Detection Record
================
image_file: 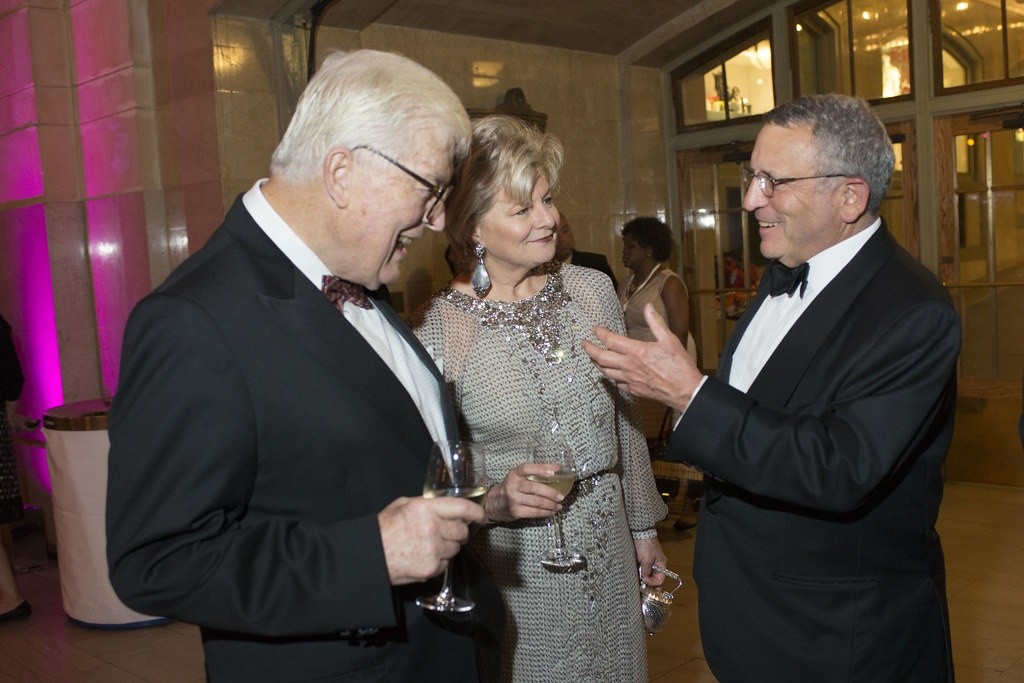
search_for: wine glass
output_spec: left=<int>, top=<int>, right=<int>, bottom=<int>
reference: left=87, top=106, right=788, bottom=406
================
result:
left=525, top=436, right=583, bottom=566
left=415, top=439, right=487, bottom=612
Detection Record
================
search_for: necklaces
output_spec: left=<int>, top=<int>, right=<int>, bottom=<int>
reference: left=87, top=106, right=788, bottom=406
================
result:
left=630, top=283, right=636, bottom=292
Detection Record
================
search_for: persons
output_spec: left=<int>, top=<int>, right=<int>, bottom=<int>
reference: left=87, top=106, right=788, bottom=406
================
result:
left=106, top=49, right=505, bottom=683
left=556, top=211, right=618, bottom=291
left=582, top=94, right=961, bottom=683
left=0, top=312, right=40, bottom=623
left=620, top=217, right=697, bottom=440
left=408, top=114, right=660, bottom=683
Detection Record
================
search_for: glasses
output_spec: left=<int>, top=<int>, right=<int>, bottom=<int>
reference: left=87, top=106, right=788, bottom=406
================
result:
left=349, top=145, right=454, bottom=223
left=740, top=165, right=851, bottom=198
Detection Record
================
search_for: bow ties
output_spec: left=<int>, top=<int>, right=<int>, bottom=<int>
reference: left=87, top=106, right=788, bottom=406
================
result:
left=322, top=275, right=374, bottom=313
left=769, top=262, right=808, bottom=299
